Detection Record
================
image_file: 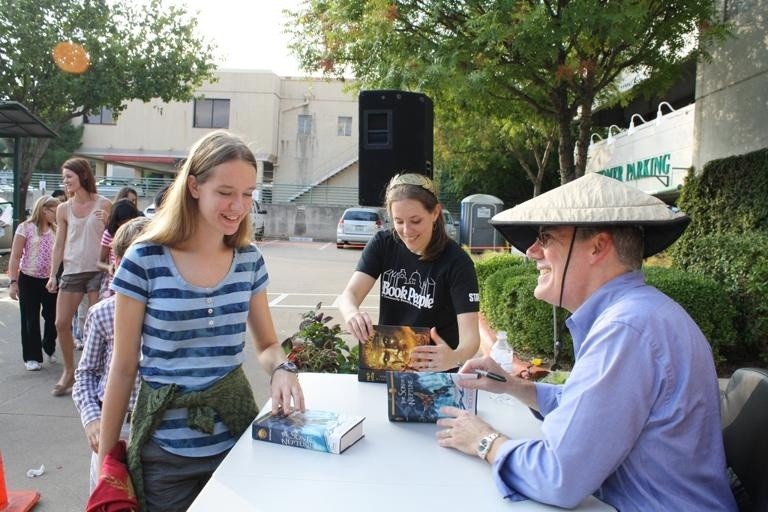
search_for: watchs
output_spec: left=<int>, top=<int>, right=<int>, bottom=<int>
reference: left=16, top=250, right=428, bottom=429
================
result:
left=476, top=433, right=499, bottom=462
left=269, top=362, right=297, bottom=383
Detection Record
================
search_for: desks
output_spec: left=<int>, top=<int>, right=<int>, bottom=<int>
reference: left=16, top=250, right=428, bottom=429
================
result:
left=182, top=370, right=621, bottom=511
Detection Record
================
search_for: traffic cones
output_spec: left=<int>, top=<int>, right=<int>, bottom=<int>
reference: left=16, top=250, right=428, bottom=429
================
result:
left=0, top=447, right=44, bottom=512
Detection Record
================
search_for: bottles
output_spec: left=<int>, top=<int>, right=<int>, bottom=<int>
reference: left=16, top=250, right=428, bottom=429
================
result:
left=489, top=331, right=515, bottom=404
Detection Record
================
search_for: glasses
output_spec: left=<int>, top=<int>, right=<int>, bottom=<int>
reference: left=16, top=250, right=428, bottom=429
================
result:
left=536, top=227, right=600, bottom=248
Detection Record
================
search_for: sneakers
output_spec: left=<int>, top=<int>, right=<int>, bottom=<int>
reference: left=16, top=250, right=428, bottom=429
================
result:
left=23, top=338, right=85, bottom=371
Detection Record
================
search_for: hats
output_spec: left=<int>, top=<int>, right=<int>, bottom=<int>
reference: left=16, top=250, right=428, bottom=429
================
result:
left=488, top=170, right=691, bottom=258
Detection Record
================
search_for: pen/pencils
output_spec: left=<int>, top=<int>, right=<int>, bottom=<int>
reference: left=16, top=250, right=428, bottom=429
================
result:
left=456, top=362, right=507, bottom=382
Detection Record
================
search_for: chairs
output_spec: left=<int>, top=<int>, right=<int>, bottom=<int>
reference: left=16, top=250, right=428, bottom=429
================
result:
left=715, top=366, right=766, bottom=511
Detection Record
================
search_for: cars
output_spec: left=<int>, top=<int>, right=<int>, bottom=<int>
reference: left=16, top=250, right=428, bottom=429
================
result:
left=0, top=197, right=33, bottom=255
left=335, top=206, right=388, bottom=250
left=0, top=183, right=38, bottom=192
left=143, top=200, right=157, bottom=219
left=440, top=208, right=459, bottom=243
left=95, top=178, right=143, bottom=195
left=249, top=200, right=269, bottom=240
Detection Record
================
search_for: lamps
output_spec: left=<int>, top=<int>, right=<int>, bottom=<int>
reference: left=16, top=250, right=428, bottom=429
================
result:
left=586, top=100, right=692, bottom=150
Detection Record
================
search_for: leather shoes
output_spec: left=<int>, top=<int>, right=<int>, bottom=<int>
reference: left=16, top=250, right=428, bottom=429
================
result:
left=51, top=377, right=76, bottom=395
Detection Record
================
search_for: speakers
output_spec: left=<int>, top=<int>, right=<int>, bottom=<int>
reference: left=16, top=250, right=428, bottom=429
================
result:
left=359, top=90, right=434, bottom=207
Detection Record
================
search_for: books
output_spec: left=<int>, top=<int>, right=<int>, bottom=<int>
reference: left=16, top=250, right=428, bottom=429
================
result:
left=252, top=406, right=366, bottom=455
left=357, top=324, right=432, bottom=384
left=385, top=372, right=477, bottom=422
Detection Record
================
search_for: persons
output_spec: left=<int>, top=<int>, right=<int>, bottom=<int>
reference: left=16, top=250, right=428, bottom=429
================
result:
left=70, top=217, right=151, bottom=493
left=8, top=156, right=168, bottom=396
left=435, top=171, right=738, bottom=512
left=98, top=130, right=306, bottom=511
left=334, top=173, right=481, bottom=374
left=362, top=326, right=430, bottom=373
